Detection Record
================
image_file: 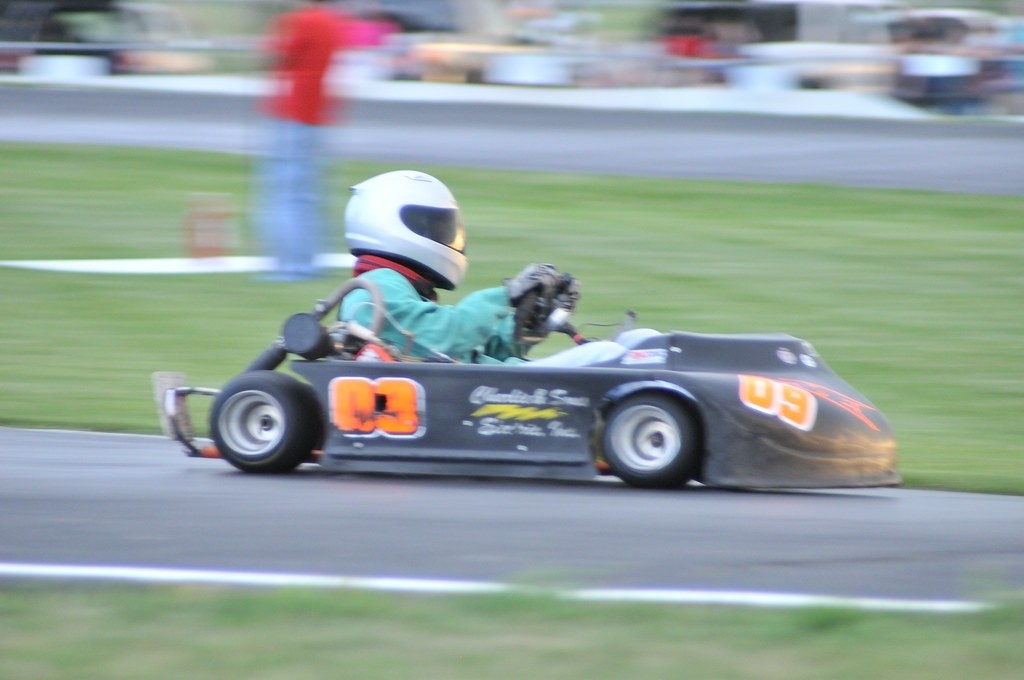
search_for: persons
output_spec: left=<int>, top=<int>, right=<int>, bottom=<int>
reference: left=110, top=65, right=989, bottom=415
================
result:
left=252, top=0, right=397, bottom=281
left=335, top=169, right=581, bottom=365
left=874, top=16, right=1024, bottom=115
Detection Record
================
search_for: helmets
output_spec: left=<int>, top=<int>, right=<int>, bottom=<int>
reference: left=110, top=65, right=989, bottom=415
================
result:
left=342, top=167, right=472, bottom=292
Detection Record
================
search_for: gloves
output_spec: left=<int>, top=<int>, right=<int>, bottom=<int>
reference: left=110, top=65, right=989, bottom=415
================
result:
left=505, top=261, right=581, bottom=309
